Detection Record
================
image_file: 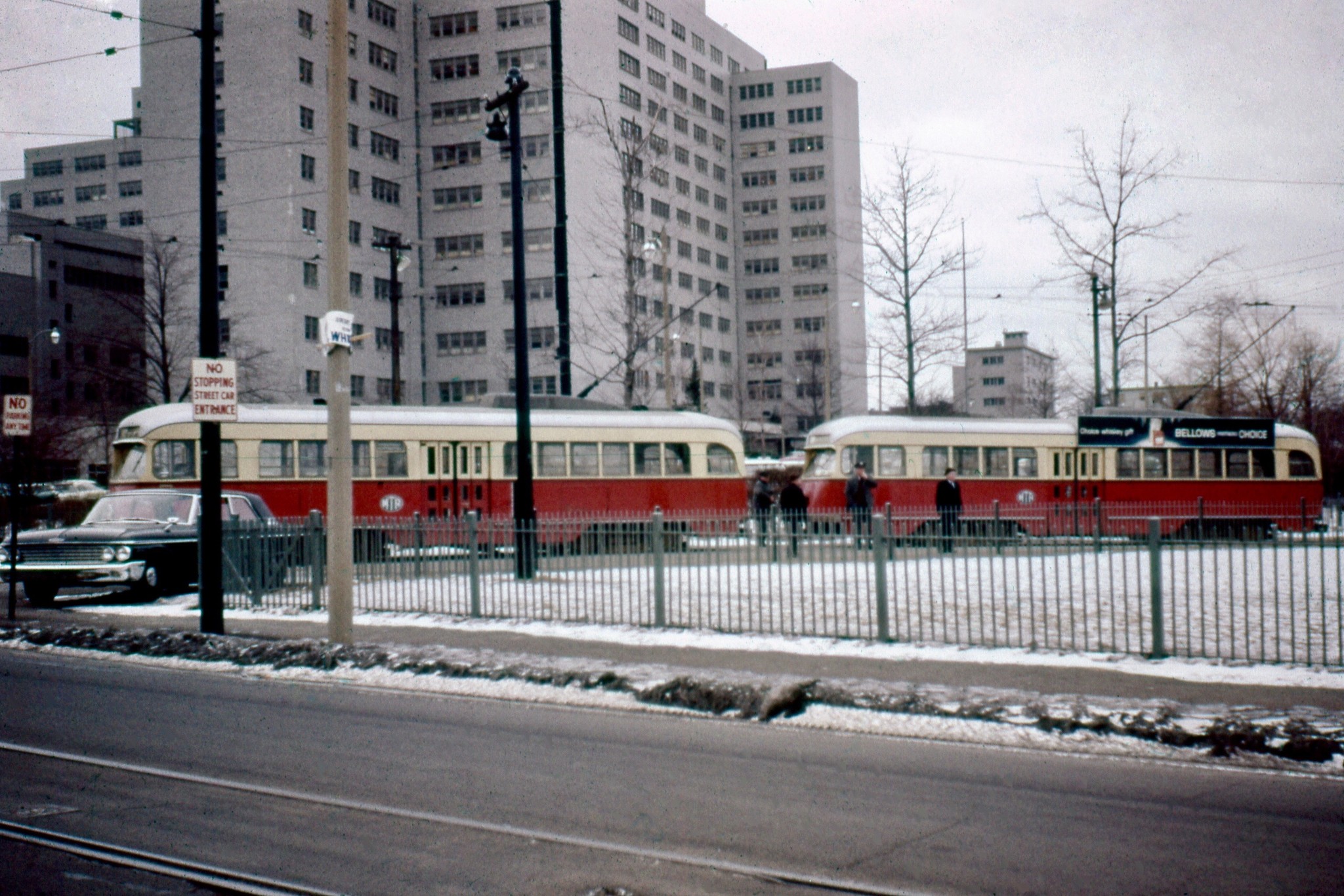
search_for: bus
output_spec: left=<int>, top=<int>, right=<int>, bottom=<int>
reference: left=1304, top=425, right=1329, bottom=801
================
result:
left=793, top=413, right=1329, bottom=546
left=97, top=400, right=755, bottom=563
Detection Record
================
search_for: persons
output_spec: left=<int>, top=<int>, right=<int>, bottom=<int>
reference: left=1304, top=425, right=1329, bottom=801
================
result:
left=845, top=462, right=877, bottom=551
left=752, top=472, right=776, bottom=547
left=936, top=468, right=962, bottom=552
left=779, top=473, right=807, bottom=555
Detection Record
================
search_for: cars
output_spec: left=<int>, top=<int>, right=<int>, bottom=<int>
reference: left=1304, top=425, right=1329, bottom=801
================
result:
left=0, top=485, right=285, bottom=606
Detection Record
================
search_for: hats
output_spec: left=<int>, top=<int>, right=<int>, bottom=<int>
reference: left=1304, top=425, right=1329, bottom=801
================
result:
left=854, top=461, right=867, bottom=468
left=944, top=468, right=955, bottom=475
left=760, top=471, right=768, bottom=478
left=791, top=474, right=800, bottom=481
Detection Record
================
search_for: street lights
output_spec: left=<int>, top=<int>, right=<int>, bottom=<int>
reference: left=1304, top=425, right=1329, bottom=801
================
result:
left=480, top=64, right=542, bottom=573
left=1086, top=270, right=1118, bottom=409
